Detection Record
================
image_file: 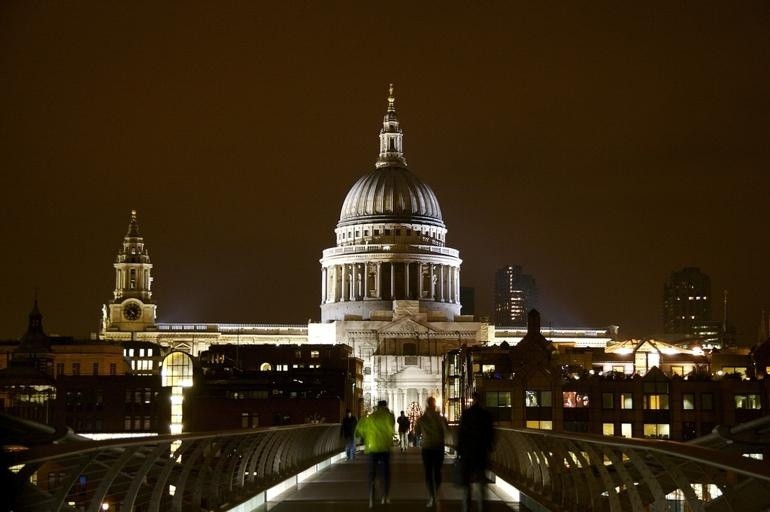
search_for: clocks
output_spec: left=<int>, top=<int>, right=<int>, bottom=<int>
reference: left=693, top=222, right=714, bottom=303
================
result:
left=123, top=302, right=142, bottom=321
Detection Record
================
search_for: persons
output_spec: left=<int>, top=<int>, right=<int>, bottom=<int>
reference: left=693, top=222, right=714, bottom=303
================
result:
left=397, top=411, right=409, bottom=453
left=409, top=426, right=420, bottom=448
left=339, top=409, right=357, bottom=464
left=271, top=411, right=283, bottom=426
left=354, top=400, right=395, bottom=508
left=456, top=392, right=497, bottom=511
left=415, top=397, right=445, bottom=511
